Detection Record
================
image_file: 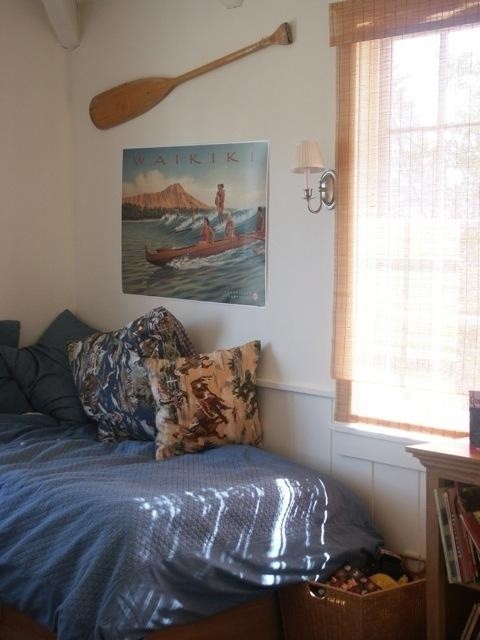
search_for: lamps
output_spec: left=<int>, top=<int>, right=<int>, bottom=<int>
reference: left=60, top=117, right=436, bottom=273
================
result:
left=291, top=140, right=335, bottom=215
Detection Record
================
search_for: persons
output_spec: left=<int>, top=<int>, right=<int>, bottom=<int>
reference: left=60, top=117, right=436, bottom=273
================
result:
left=164, top=183, right=234, bottom=247
left=256, top=206, right=265, bottom=233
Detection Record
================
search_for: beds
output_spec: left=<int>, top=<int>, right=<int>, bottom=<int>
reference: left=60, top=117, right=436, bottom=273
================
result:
left=0, top=412, right=383, bottom=640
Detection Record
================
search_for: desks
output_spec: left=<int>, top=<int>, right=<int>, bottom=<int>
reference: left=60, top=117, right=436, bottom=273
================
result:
left=406, top=437, right=480, bottom=639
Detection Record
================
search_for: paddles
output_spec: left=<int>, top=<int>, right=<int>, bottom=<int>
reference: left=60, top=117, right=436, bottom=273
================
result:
left=89, top=22, right=293, bottom=130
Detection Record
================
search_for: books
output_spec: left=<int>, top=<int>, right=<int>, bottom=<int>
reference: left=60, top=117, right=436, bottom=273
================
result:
left=434, top=480, right=480, bottom=640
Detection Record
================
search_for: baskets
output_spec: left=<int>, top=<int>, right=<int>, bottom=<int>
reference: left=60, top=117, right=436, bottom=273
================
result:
left=278, top=553, right=426, bottom=639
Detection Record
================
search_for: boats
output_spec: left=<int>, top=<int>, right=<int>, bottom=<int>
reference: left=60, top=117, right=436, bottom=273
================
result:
left=142, top=229, right=264, bottom=267
left=156, top=210, right=251, bottom=238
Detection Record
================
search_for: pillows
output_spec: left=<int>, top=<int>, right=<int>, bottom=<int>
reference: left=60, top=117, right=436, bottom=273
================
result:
left=0, top=309, right=106, bottom=428
left=141, top=341, right=263, bottom=459
left=64, top=307, right=197, bottom=443
left=0, top=320, right=28, bottom=416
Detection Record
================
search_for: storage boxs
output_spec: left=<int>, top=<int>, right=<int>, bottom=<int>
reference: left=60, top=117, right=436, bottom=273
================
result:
left=279, top=569, right=426, bottom=639
